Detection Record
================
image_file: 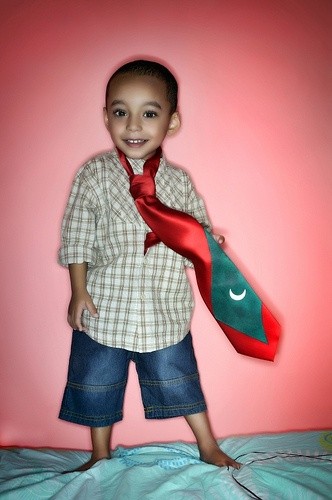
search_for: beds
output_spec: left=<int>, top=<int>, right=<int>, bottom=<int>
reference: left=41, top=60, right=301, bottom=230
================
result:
left=0, top=428, right=331, bottom=500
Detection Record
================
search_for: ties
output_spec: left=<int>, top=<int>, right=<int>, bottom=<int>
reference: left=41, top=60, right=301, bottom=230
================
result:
left=116, top=143, right=282, bottom=364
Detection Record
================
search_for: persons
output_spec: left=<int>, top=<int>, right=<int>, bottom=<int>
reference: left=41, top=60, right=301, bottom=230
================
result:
left=57, top=59, right=242, bottom=472
